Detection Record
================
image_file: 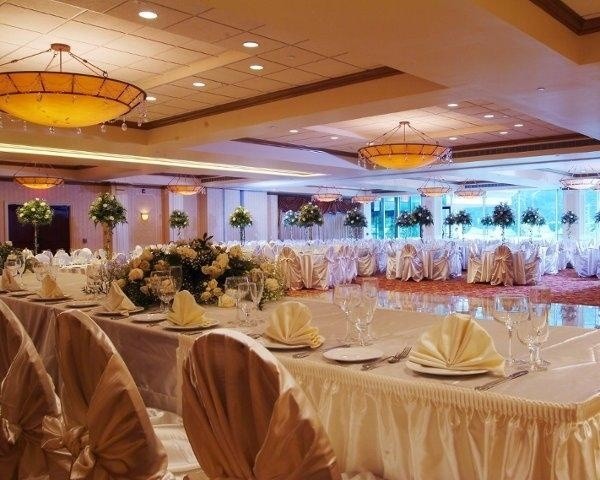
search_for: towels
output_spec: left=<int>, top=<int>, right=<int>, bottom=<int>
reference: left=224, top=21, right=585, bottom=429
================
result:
left=168, top=290, right=203, bottom=327
left=264, top=301, right=321, bottom=350
left=410, top=312, right=506, bottom=379
left=28, top=276, right=66, bottom=299
left=96, top=280, right=136, bottom=310
left=0, top=269, right=21, bottom=293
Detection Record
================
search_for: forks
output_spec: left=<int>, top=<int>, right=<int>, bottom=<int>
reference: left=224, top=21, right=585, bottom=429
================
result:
left=362, top=345, right=412, bottom=365
left=360, top=352, right=402, bottom=371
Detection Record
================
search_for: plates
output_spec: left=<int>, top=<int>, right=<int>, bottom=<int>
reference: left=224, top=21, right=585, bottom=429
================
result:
left=93, top=305, right=145, bottom=316
left=65, top=300, right=103, bottom=308
left=257, top=333, right=326, bottom=349
left=402, top=357, right=505, bottom=375
left=323, top=347, right=384, bottom=362
left=129, top=313, right=170, bottom=323
left=158, top=317, right=220, bottom=331
left=5, top=288, right=39, bottom=297
left=26, top=294, right=73, bottom=302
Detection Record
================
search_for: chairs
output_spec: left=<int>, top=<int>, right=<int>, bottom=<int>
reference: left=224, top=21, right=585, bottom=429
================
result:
left=42, top=309, right=201, bottom=479
left=182, top=329, right=382, bottom=480
left=23, top=244, right=147, bottom=278
left=1, top=301, right=61, bottom=480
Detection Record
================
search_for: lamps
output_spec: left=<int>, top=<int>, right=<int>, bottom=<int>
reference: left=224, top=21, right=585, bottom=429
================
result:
left=352, top=188, right=379, bottom=204
left=310, top=187, right=343, bottom=203
left=140, top=212, right=149, bottom=221
left=13, top=158, right=64, bottom=190
left=454, top=178, right=486, bottom=201
left=165, top=175, right=204, bottom=196
left=357, top=121, right=452, bottom=172
left=417, top=176, right=451, bottom=197
left=0, top=44, right=148, bottom=136
left=558, top=163, right=600, bottom=189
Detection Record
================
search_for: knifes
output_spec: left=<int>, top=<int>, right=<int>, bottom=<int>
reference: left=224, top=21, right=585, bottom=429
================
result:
left=474, top=370, right=528, bottom=392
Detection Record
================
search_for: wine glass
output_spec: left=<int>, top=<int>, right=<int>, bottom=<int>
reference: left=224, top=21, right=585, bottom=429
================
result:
left=101, top=261, right=115, bottom=296
left=361, top=278, right=379, bottom=340
left=333, top=284, right=353, bottom=345
left=87, top=266, right=102, bottom=299
left=354, top=294, right=375, bottom=346
left=492, top=292, right=526, bottom=375
left=237, top=282, right=260, bottom=336
left=16, top=254, right=28, bottom=279
left=223, top=276, right=249, bottom=329
left=157, top=276, right=176, bottom=316
left=347, top=284, right=361, bottom=342
left=528, top=301, right=549, bottom=366
left=150, top=270, right=169, bottom=313
left=247, top=271, right=266, bottom=320
left=515, top=306, right=542, bottom=370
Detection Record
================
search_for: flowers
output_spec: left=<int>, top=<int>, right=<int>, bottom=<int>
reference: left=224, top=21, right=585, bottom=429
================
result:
left=88, top=235, right=287, bottom=306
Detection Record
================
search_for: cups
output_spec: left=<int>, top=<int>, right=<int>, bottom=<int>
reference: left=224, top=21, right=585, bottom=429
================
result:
left=3, top=259, right=18, bottom=277
left=35, top=260, right=48, bottom=281
left=169, top=265, right=183, bottom=292
